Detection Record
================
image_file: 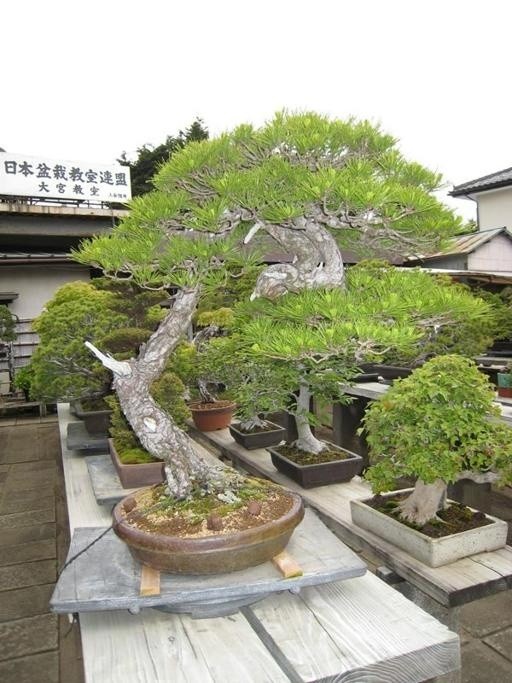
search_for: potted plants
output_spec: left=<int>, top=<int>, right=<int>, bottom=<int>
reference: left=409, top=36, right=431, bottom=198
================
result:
left=175, top=308, right=238, bottom=431
left=349, top=353, right=512, bottom=568
left=85, top=106, right=480, bottom=589
left=232, top=351, right=365, bottom=486
left=227, top=370, right=287, bottom=448
left=102, top=341, right=184, bottom=486
left=10, top=277, right=129, bottom=432
